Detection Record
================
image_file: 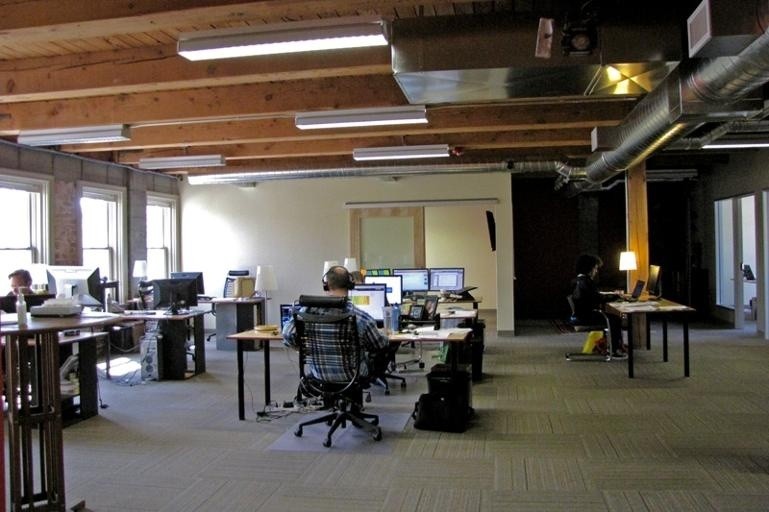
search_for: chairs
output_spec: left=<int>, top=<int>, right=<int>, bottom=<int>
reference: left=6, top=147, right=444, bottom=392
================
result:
left=565, top=294, right=612, bottom=362
left=207, top=271, right=249, bottom=341
left=294, top=295, right=389, bottom=447
left=139, top=281, right=196, bottom=361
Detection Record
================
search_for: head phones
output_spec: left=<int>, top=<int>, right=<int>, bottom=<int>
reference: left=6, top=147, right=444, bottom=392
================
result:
left=322, top=270, right=355, bottom=291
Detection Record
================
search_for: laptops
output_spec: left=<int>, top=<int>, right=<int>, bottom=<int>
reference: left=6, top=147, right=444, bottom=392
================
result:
left=744, top=265, right=756, bottom=280
left=623, top=279, right=645, bottom=302
left=280, top=304, right=301, bottom=331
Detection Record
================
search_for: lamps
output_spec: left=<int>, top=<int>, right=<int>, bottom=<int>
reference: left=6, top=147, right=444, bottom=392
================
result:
left=702, top=143, right=769, bottom=149
left=295, top=105, right=429, bottom=130
left=138, top=154, right=226, bottom=170
left=133, top=260, right=146, bottom=286
left=323, top=261, right=338, bottom=282
left=618, top=251, right=638, bottom=294
left=255, top=265, right=278, bottom=325
left=352, top=143, right=451, bottom=161
left=344, top=258, right=357, bottom=273
left=176, top=15, right=390, bottom=64
left=17, top=124, right=130, bottom=147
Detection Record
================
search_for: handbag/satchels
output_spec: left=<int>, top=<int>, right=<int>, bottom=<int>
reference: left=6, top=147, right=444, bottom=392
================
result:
left=413, top=390, right=468, bottom=433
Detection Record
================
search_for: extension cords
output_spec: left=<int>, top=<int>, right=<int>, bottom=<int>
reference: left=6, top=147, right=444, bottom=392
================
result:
left=263, top=405, right=300, bottom=412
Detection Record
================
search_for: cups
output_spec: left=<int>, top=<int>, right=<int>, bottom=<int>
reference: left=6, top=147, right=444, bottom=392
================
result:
left=439, top=288, right=447, bottom=301
left=440, top=289, right=447, bottom=294
left=382, top=306, right=393, bottom=336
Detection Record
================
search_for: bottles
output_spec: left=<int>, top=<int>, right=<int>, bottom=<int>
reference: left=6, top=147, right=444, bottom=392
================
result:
left=15, top=294, right=27, bottom=326
left=107, top=293, right=111, bottom=312
left=392, top=302, right=400, bottom=331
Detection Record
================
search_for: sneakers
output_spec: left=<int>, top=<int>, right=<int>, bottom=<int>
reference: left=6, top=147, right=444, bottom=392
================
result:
left=606, top=349, right=629, bottom=361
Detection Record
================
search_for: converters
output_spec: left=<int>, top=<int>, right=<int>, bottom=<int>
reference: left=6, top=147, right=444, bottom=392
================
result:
left=283, top=401, right=294, bottom=407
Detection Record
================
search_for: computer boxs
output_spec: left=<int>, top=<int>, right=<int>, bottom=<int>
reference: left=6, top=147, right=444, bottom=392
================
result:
left=458, top=322, right=485, bottom=370
left=431, top=363, right=473, bottom=408
left=427, top=371, right=470, bottom=406
left=104, top=325, right=133, bottom=354
left=139, top=337, right=187, bottom=382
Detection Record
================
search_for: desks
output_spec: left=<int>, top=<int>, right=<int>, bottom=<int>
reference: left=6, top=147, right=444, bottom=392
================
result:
left=599, top=290, right=696, bottom=378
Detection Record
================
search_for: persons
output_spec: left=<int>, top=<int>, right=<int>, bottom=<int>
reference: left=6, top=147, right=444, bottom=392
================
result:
left=3, top=270, right=40, bottom=312
left=573, top=253, right=627, bottom=359
left=283, top=266, right=390, bottom=402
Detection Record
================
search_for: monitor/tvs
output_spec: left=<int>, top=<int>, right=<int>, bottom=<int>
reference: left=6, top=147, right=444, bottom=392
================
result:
left=430, top=267, right=464, bottom=300
left=348, top=283, right=387, bottom=328
left=170, top=271, right=205, bottom=300
left=0, top=294, right=55, bottom=314
left=153, top=279, right=198, bottom=315
left=87, top=268, right=104, bottom=308
left=392, top=269, right=430, bottom=301
left=646, top=264, right=663, bottom=300
left=486, top=210, right=495, bottom=251
left=366, top=269, right=391, bottom=277
left=364, top=275, right=403, bottom=306
